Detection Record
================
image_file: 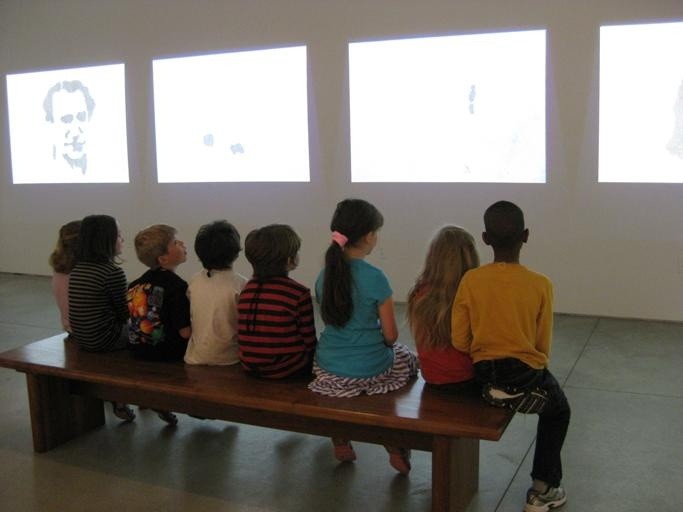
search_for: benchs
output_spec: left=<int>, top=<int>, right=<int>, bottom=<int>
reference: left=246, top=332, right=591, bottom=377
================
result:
left=0, top=321, right=519, bottom=511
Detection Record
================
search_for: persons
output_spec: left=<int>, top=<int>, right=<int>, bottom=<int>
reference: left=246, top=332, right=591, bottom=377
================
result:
left=305, top=198, right=418, bottom=477
left=124, top=223, right=194, bottom=363
left=235, top=222, right=317, bottom=382
left=450, top=200, right=570, bottom=512
left=403, top=224, right=481, bottom=395
left=47, top=218, right=87, bottom=336
left=41, top=78, right=97, bottom=175
left=67, top=213, right=179, bottom=428
left=183, top=217, right=250, bottom=421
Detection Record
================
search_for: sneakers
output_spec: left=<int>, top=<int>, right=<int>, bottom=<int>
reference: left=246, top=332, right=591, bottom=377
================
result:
left=156, top=410, right=177, bottom=425
left=525, top=487, right=567, bottom=512
left=385, top=444, right=411, bottom=474
left=332, top=436, right=356, bottom=464
left=113, top=405, right=135, bottom=421
